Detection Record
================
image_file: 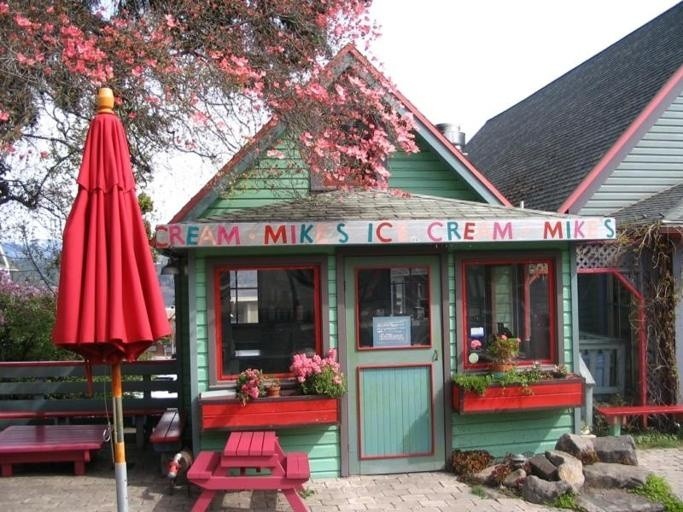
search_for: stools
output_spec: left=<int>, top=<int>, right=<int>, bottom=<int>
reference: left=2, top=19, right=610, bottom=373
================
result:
left=147, top=407, right=186, bottom=476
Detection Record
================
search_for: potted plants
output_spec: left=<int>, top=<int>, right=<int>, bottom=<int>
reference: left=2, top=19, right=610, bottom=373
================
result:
left=266, top=378, right=281, bottom=395
left=451, top=370, right=585, bottom=416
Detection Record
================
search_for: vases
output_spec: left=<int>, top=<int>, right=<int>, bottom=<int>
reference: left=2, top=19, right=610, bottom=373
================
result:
left=495, top=361, right=513, bottom=371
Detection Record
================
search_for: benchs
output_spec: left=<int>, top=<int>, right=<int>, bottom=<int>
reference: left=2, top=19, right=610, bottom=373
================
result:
left=186, top=451, right=223, bottom=490
left=0, top=360, right=180, bottom=448
left=282, top=452, right=309, bottom=490
left=594, top=406, right=682, bottom=436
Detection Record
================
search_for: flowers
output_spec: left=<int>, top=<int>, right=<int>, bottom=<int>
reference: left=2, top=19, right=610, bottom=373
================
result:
left=234, top=369, right=265, bottom=407
left=488, top=334, right=521, bottom=363
left=288, top=349, right=345, bottom=398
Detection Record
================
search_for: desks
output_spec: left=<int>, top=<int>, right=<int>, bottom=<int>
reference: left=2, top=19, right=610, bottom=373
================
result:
left=0, top=425, right=111, bottom=476
left=190, top=432, right=308, bottom=512
left=232, top=348, right=291, bottom=371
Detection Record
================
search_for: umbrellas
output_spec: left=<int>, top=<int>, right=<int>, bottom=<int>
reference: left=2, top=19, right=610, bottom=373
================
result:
left=54, top=80, right=174, bottom=512
